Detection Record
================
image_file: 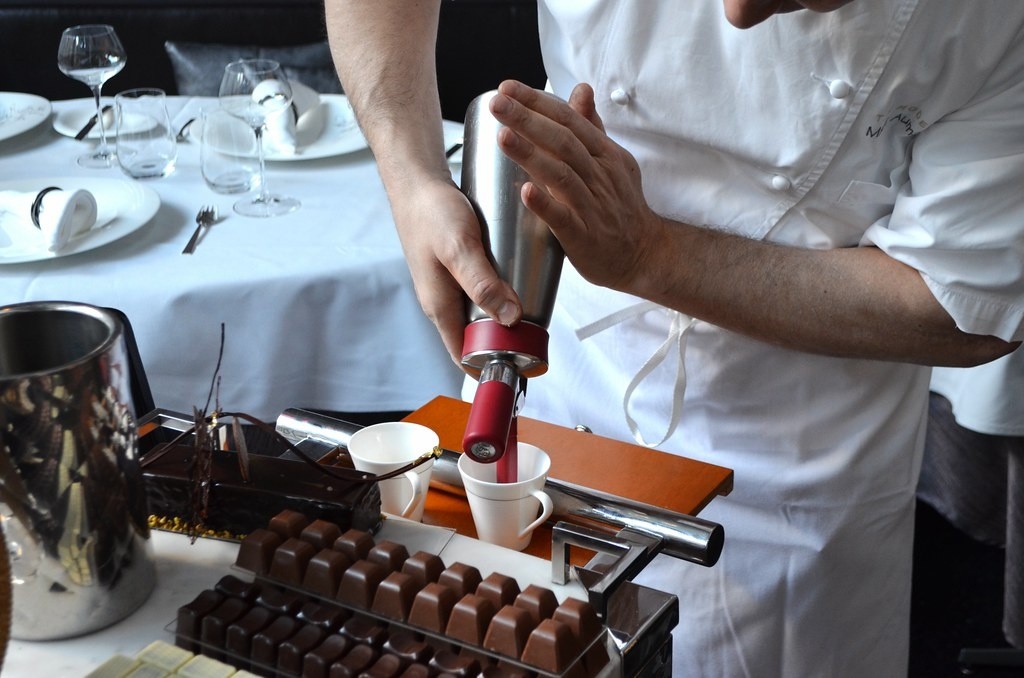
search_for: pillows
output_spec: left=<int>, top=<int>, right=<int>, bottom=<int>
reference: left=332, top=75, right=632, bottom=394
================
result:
left=161, top=35, right=348, bottom=97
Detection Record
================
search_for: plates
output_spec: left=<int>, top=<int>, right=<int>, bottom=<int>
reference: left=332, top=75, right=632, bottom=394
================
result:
left=190, top=94, right=369, bottom=161
left=52, top=97, right=158, bottom=138
left=0, top=92, right=52, bottom=141
left=0, top=175, right=161, bottom=264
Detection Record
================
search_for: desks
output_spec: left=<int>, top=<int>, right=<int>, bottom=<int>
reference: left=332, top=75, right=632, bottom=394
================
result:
left=0, top=98, right=467, bottom=426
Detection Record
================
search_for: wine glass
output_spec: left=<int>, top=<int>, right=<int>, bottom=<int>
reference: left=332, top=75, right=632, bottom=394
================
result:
left=219, top=59, right=301, bottom=218
left=57, top=24, right=127, bottom=168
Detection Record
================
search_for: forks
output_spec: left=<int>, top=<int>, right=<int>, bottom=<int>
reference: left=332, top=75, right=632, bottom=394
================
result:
left=176, top=119, right=195, bottom=142
left=181, top=205, right=214, bottom=253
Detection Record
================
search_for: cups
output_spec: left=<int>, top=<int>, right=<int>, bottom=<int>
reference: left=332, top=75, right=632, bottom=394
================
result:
left=458, top=441, right=554, bottom=551
left=199, top=100, right=262, bottom=195
left=346, top=422, right=441, bottom=523
left=1, top=301, right=156, bottom=642
left=114, top=88, right=178, bottom=180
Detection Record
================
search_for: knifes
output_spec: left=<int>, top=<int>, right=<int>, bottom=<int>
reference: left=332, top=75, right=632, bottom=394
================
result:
left=74, top=105, right=113, bottom=141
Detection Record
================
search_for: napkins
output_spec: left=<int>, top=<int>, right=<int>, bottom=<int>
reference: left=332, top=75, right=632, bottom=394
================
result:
left=0, top=189, right=99, bottom=257
left=252, top=78, right=326, bottom=158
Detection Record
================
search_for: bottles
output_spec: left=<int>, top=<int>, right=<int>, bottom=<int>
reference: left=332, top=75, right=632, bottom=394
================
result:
left=459, top=88, right=565, bottom=464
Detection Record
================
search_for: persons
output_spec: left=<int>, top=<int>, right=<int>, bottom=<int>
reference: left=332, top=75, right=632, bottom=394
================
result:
left=325, top=0, right=1024, bottom=678
